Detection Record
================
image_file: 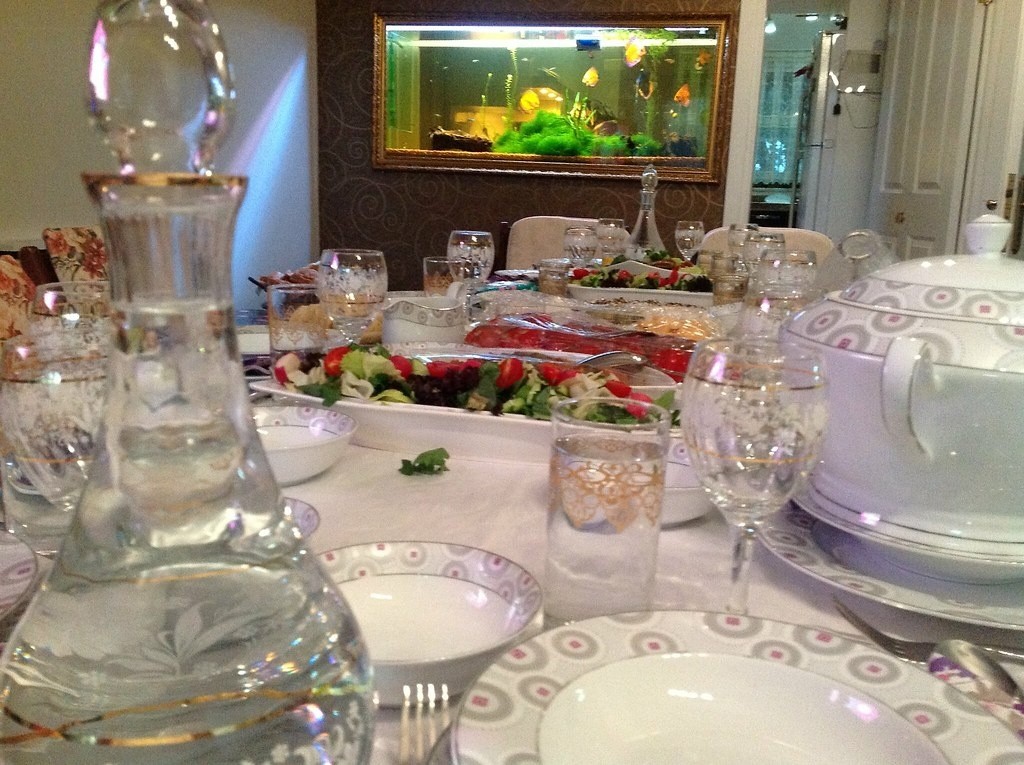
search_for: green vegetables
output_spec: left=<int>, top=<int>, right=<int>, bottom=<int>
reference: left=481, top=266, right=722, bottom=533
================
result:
left=570, top=249, right=714, bottom=293
left=294, top=340, right=681, bottom=475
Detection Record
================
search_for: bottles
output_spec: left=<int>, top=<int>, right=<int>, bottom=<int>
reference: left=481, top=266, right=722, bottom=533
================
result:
left=626, top=164, right=666, bottom=261
left=2, top=0, right=376, bottom=764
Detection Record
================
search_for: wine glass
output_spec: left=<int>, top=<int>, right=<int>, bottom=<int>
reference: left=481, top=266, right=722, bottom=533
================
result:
left=678, top=336, right=833, bottom=611
left=0, top=218, right=898, bottom=560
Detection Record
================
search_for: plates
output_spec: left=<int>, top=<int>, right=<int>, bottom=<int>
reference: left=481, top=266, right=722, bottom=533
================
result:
left=753, top=503, right=1023, bottom=631
left=752, top=498, right=1024, bottom=633
left=0, top=269, right=801, bottom=619
left=787, top=489, right=1023, bottom=586
left=452, top=608, right=1022, bottom=764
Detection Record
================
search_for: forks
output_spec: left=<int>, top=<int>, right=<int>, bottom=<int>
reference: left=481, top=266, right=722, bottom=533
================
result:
left=398, top=682, right=450, bottom=764
left=835, top=601, right=1024, bottom=660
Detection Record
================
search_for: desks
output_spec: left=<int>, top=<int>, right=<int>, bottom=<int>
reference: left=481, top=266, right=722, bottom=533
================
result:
left=0, top=289, right=1024, bottom=765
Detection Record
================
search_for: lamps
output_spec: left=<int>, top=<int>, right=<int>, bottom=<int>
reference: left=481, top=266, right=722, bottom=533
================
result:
left=805, top=13, right=820, bottom=22
left=764, top=15, right=777, bottom=33
left=828, top=13, right=847, bottom=30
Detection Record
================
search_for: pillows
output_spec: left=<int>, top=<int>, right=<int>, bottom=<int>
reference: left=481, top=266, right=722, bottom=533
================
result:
left=0, top=254, right=52, bottom=373
left=42, top=224, right=113, bottom=360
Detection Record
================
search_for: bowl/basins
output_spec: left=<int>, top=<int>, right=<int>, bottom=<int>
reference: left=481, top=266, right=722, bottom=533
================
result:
left=283, top=496, right=321, bottom=537
left=309, top=542, right=542, bottom=705
left=661, top=437, right=716, bottom=526
left=236, top=284, right=710, bottom=489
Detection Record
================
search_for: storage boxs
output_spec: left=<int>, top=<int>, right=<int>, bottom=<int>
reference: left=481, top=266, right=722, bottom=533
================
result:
left=364, top=9, right=732, bottom=187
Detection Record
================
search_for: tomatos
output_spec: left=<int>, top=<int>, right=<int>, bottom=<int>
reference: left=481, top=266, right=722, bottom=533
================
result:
left=322, top=311, right=714, bottom=411
left=619, top=270, right=632, bottom=278
left=573, top=268, right=589, bottom=276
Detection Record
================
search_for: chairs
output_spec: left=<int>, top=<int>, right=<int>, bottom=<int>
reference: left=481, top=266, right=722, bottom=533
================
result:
left=690, top=227, right=834, bottom=287
left=21, top=245, right=58, bottom=288
left=496, top=215, right=636, bottom=271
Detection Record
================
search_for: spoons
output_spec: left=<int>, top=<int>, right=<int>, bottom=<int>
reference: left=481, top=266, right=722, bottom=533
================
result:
left=926, top=638, right=1024, bottom=714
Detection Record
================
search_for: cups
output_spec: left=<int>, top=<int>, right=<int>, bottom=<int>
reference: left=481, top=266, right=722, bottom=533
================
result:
left=542, top=399, right=676, bottom=627
left=266, top=254, right=750, bottom=363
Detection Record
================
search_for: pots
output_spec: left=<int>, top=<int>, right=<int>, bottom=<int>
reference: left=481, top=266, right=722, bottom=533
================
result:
left=784, top=211, right=1024, bottom=512
